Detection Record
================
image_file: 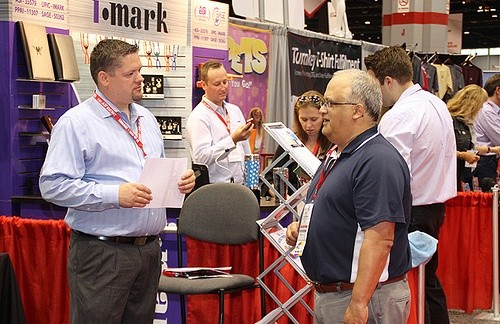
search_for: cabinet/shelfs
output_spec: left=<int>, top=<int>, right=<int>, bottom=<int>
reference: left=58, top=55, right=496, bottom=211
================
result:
left=17, top=78, right=70, bottom=188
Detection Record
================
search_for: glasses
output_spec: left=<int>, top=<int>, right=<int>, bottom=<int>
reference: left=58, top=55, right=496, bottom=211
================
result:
left=320, top=100, right=357, bottom=108
left=298, top=96, right=320, bottom=103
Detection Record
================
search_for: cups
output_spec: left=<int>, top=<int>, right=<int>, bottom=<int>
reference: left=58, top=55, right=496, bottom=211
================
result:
left=274, top=168, right=288, bottom=203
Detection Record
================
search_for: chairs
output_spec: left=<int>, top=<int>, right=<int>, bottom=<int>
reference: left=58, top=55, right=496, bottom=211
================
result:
left=158, top=182, right=267, bottom=324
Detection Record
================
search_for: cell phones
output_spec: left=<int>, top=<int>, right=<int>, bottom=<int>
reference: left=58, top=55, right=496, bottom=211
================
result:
left=247, top=118, right=254, bottom=128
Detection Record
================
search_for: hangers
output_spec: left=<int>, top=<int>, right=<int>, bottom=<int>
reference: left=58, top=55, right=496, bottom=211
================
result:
left=412, top=50, right=473, bottom=66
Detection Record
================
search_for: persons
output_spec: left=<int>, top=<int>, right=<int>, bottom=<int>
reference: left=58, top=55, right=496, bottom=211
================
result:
left=286, top=69, right=412, bottom=324
left=261, top=90, right=334, bottom=198
left=364, top=46, right=457, bottom=324
left=447, top=73, right=500, bottom=192
left=185, top=59, right=255, bottom=184
left=39, top=39, right=196, bottom=324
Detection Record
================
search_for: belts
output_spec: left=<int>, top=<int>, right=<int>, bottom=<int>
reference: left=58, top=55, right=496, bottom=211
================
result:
left=72, top=228, right=158, bottom=246
left=314, top=273, right=406, bottom=293
